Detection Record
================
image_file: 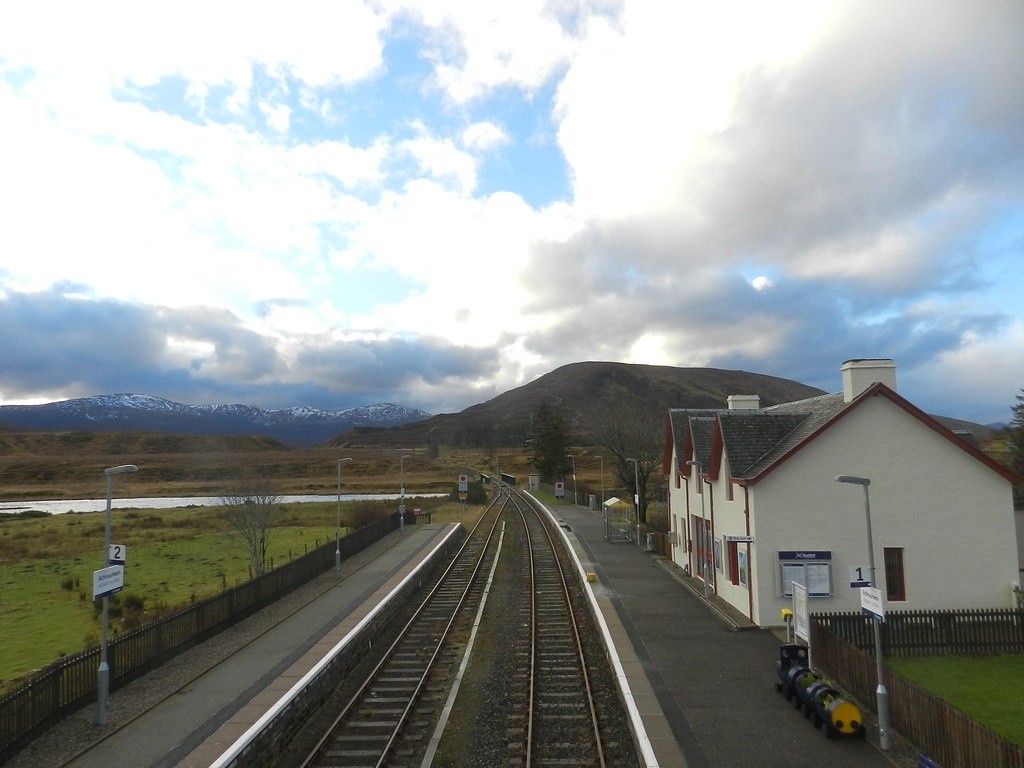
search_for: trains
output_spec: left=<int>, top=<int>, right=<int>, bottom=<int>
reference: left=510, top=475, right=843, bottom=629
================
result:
left=773, top=608, right=867, bottom=743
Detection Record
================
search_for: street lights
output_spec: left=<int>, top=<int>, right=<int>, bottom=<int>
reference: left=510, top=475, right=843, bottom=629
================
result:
left=627, top=457, right=641, bottom=546
left=333, top=457, right=355, bottom=577
left=398, top=455, right=411, bottom=532
left=97, top=464, right=138, bottom=726
left=567, top=454, right=578, bottom=504
left=834, top=475, right=894, bottom=753
left=594, top=455, right=605, bottom=521
